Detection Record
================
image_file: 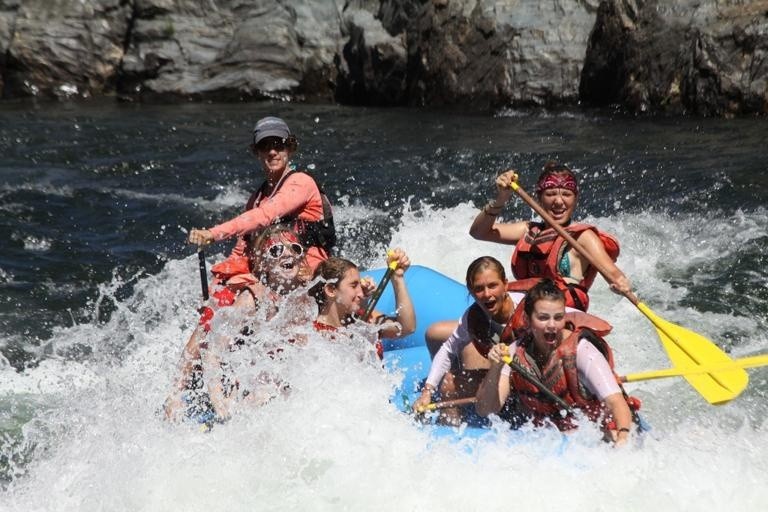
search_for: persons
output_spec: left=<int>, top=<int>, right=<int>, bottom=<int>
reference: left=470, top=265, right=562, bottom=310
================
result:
left=191, top=116, right=331, bottom=282
left=303, top=249, right=417, bottom=366
left=162, top=254, right=250, bottom=424
left=425, top=166, right=632, bottom=363
left=415, top=256, right=580, bottom=432
left=200, top=224, right=377, bottom=421
left=475, top=279, right=633, bottom=450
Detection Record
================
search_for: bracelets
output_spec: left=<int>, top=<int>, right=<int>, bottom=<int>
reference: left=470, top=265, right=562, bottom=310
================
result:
left=619, top=428, right=629, bottom=433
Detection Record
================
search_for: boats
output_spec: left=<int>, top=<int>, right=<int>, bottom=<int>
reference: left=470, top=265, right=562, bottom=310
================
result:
left=346, top=265, right=651, bottom=454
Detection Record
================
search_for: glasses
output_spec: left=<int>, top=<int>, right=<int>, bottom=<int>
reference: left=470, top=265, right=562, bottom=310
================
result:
left=257, top=144, right=288, bottom=151
left=265, top=242, right=306, bottom=259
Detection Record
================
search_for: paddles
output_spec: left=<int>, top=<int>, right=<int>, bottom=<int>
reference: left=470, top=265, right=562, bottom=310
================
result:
left=418, top=353, right=768, bottom=415
left=510, top=173, right=749, bottom=405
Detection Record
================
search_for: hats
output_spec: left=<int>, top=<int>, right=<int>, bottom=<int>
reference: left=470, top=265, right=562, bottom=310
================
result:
left=254, top=117, right=294, bottom=144
